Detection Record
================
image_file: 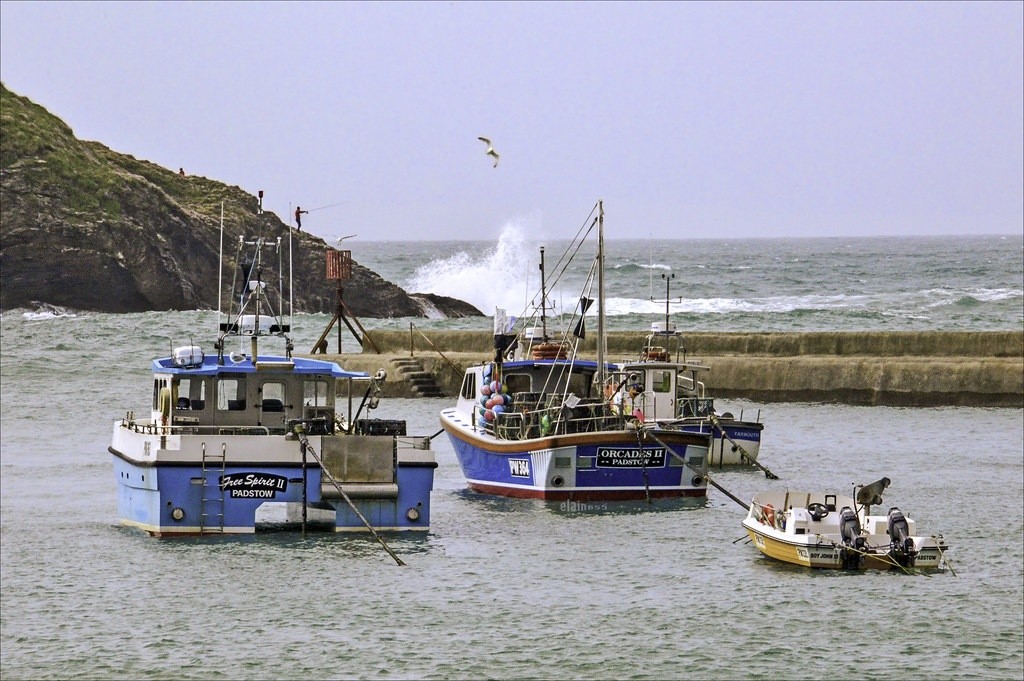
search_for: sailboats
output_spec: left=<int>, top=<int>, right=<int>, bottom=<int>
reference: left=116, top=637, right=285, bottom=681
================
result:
left=439, top=199, right=713, bottom=502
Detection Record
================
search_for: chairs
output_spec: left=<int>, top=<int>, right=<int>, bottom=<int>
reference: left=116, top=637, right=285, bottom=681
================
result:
left=177, top=397, right=205, bottom=410
left=228, top=400, right=245, bottom=410
left=262, top=399, right=284, bottom=412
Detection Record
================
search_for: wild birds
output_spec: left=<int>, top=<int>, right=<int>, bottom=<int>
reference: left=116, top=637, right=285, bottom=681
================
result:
left=476, top=136, right=501, bottom=169
left=322, top=232, right=358, bottom=246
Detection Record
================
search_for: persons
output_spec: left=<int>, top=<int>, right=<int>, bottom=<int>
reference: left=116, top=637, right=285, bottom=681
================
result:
left=295, top=206, right=308, bottom=234
left=179, top=168, right=184, bottom=176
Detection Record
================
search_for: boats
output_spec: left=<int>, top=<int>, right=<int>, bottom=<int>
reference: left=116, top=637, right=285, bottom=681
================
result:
left=612, top=274, right=765, bottom=466
left=741, top=482, right=950, bottom=569
left=107, top=190, right=445, bottom=538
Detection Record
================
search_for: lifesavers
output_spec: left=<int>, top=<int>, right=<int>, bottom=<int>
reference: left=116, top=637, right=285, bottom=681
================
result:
left=763, top=503, right=774, bottom=526
left=642, top=350, right=672, bottom=362
left=530, top=342, right=567, bottom=361
left=159, top=388, right=170, bottom=418
